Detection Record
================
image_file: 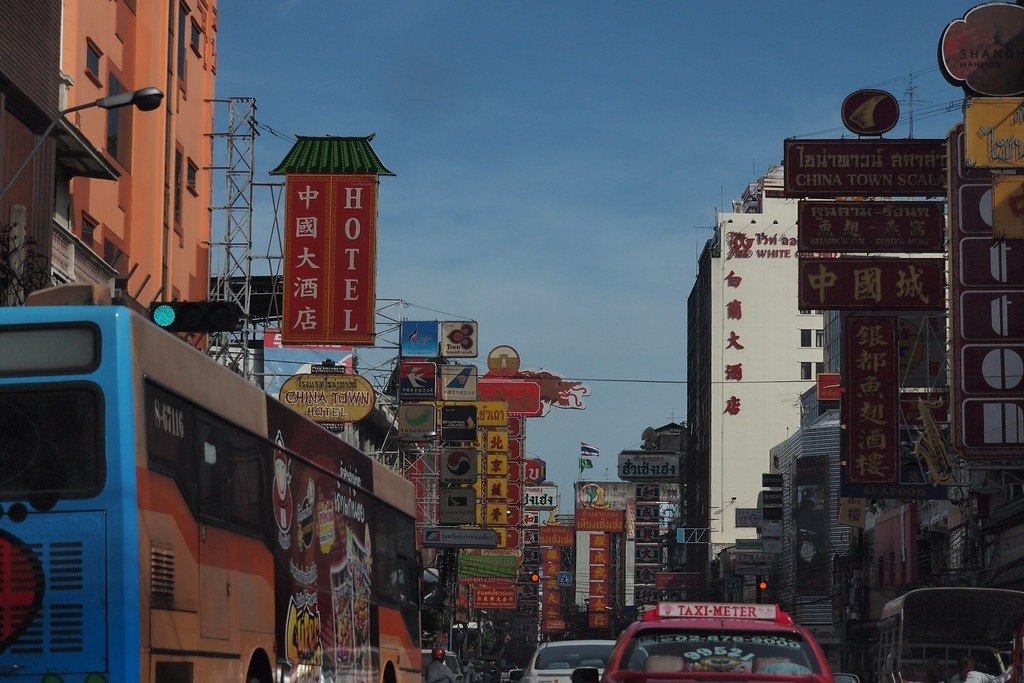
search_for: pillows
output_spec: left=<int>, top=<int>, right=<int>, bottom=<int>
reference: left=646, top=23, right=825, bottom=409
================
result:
left=755, top=657, right=790, bottom=675
left=644, top=655, right=684, bottom=672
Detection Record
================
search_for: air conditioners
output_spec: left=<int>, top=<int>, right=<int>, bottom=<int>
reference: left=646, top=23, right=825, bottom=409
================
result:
left=1005, top=483, right=1023, bottom=501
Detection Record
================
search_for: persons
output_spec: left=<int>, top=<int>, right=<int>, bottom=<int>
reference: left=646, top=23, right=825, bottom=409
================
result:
left=949, top=655, right=1016, bottom=683
left=425, top=647, right=457, bottom=683
left=925, top=655, right=949, bottom=683
left=462, top=656, right=474, bottom=683
left=486, top=661, right=497, bottom=683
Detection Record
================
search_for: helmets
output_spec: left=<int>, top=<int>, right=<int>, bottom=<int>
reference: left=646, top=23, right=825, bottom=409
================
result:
left=432, top=648, right=445, bottom=659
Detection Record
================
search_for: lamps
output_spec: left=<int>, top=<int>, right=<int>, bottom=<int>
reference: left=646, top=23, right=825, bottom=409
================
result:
left=719, top=219, right=733, bottom=230
left=739, top=220, right=756, bottom=233
left=785, top=220, right=798, bottom=232
left=764, top=220, right=778, bottom=232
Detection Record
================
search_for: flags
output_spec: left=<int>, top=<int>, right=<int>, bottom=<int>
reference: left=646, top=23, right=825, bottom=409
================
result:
left=581, top=442, right=600, bottom=457
left=579, top=459, right=593, bottom=469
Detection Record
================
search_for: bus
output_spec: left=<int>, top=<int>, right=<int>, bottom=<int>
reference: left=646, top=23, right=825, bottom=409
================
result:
left=451, top=621, right=494, bottom=661
left=0, top=304, right=423, bottom=683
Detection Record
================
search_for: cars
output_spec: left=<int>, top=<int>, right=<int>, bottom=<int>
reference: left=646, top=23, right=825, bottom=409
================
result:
left=510, top=639, right=648, bottom=683
left=601, top=601, right=837, bottom=683
left=501, top=665, right=519, bottom=682
left=458, top=656, right=500, bottom=683
left=833, top=673, right=861, bottom=683
left=421, top=649, right=464, bottom=683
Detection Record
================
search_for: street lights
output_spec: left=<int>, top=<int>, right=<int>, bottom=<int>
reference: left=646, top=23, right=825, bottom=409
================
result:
left=0, top=86, right=164, bottom=201
left=800, top=527, right=844, bottom=674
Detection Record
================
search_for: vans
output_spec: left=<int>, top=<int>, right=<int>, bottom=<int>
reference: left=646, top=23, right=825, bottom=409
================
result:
left=877, top=587, right=1024, bottom=683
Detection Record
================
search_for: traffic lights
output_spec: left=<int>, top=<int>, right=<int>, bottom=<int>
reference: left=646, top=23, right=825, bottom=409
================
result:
left=150, top=301, right=238, bottom=332
left=759, top=582, right=767, bottom=589
left=518, top=574, right=539, bottom=583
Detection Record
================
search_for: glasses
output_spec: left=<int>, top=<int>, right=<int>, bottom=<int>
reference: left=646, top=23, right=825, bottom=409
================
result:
left=958, top=661, right=967, bottom=666
